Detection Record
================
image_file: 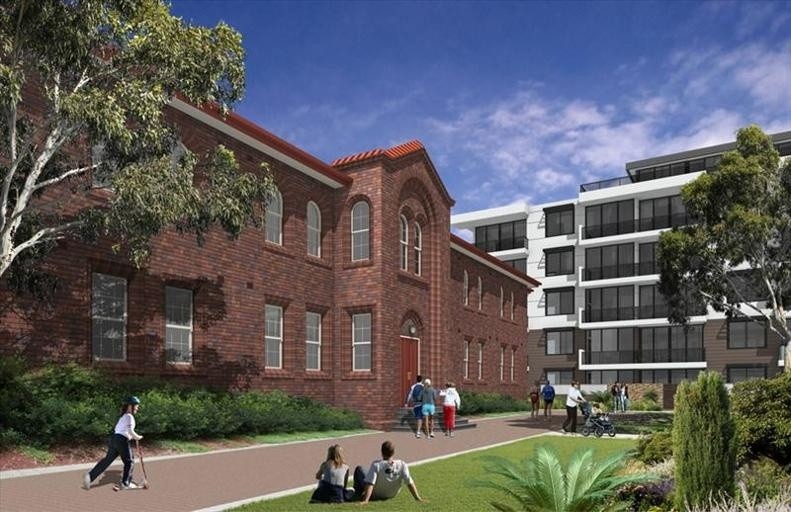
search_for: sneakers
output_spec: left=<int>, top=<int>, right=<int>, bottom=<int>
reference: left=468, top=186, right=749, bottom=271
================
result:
left=83, top=474, right=92, bottom=489
left=120, top=481, right=137, bottom=488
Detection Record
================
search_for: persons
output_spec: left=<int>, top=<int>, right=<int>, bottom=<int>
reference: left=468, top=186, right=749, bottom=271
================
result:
left=529, top=384, right=541, bottom=419
left=439, top=382, right=461, bottom=437
left=404, top=375, right=425, bottom=437
left=310, top=444, right=356, bottom=504
left=540, top=380, right=556, bottom=421
left=611, top=382, right=620, bottom=414
left=562, top=379, right=588, bottom=435
left=619, top=381, right=629, bottom=412
left=353, top=441, right=428, bottom=504
left=83, top=394, right=144, bottom=490
left=592, top=404, right=603, bottom=416
left=417, top=378, right=438, bottom=439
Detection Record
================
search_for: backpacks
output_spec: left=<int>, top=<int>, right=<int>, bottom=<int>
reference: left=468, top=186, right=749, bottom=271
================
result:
left=412, top=383, right=423, bottom=402
left=530, top=385, right=538, bottom=402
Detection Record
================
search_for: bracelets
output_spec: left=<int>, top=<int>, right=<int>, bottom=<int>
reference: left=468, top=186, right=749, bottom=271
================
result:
left=414, top=494, right=422, bottom=500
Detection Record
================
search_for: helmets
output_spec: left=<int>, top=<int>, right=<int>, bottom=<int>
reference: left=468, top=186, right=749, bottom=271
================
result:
left=128, top=396, right=139, bottom=404
